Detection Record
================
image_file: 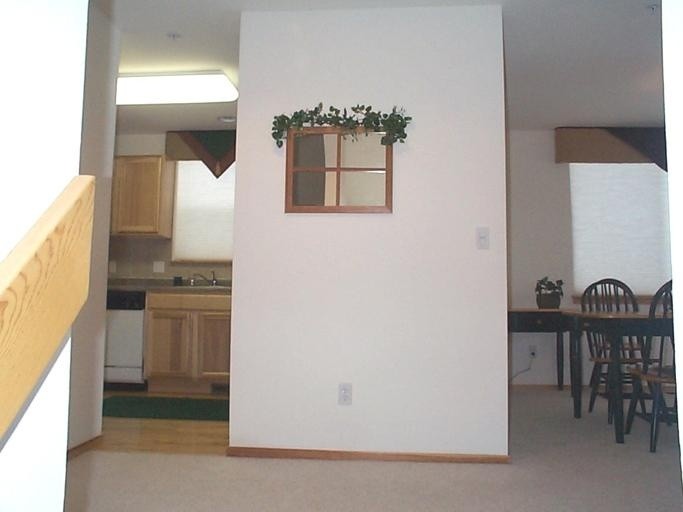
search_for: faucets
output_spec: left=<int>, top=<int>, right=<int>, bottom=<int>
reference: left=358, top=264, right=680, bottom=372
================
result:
left=191, top=269, right=217, bottom=286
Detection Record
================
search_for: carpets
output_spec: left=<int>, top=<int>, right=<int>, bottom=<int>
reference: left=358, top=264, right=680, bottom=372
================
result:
left=101, top=394, right=229, bottom=422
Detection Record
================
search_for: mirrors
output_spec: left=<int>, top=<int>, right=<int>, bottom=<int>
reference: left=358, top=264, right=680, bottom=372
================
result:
left=280, top=123, right=392, bottom=214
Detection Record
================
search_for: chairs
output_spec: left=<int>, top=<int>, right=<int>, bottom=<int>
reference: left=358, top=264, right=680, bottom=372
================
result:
left=508, top=275, right=677, bottom=455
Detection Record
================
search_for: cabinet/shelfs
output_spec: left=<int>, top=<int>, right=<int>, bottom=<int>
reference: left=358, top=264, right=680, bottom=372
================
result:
left=140, top=287, right=230, bottom=395
left=111, top=154, right=172, bottom=242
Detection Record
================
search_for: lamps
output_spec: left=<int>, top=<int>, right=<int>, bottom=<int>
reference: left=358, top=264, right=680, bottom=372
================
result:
left=116, top=71, right=236, bottom=108
